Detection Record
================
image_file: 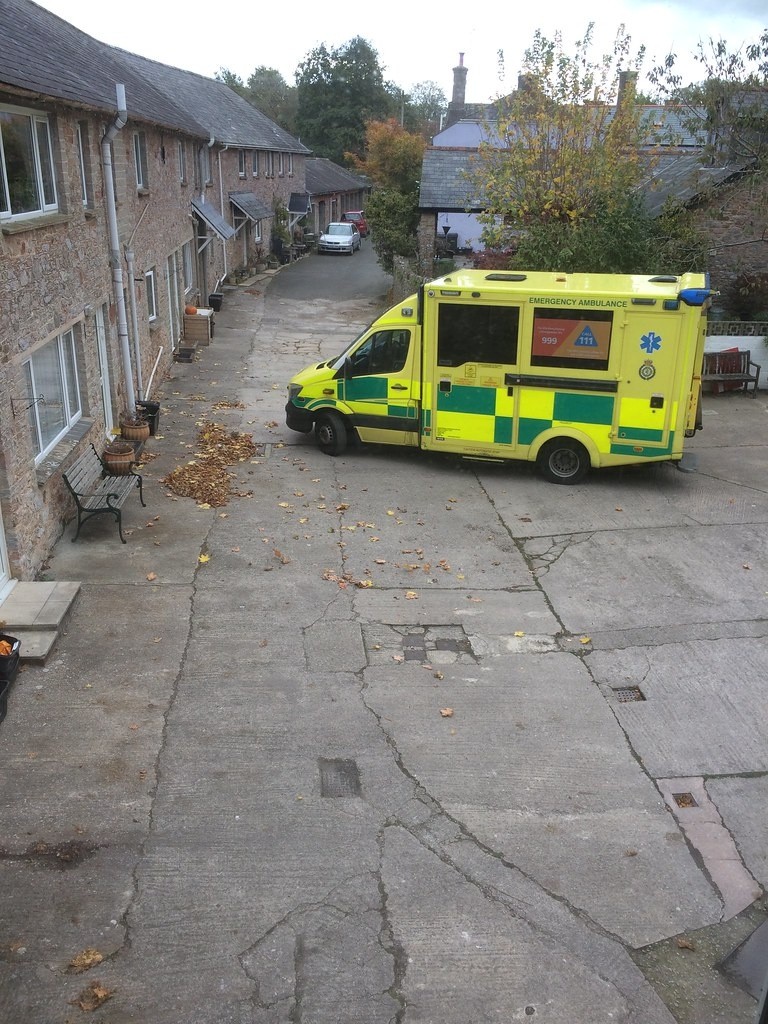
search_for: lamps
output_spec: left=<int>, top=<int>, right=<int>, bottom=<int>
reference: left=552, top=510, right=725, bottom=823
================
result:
left=439, top=213, right=451, bottom=239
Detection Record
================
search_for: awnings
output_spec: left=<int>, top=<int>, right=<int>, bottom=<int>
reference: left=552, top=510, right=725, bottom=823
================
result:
left=230, top=192, right=276, bottom=239
left=290, top=192, right=313, bottom=226
left=191, top=196, right=236, bottom=254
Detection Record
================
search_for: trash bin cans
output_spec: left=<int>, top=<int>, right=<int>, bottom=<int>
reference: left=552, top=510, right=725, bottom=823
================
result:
left=133, top=400, right=160, bottom=435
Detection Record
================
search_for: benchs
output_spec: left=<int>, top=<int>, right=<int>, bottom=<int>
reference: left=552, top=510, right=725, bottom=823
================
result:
left=701, top=350, right=761, bottom=399
left=62, top=443, right=146, bottom=544
left=305, top=241, right=315, bottom=246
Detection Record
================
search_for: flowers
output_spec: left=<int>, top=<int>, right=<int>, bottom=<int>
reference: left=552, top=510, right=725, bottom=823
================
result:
left=104, top=439, right=135, bottom=453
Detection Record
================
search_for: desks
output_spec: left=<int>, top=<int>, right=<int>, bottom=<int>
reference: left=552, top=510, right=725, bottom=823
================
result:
left=183, top=307, right=215, bottom=346
left=281, top=233, right=314, bottom=263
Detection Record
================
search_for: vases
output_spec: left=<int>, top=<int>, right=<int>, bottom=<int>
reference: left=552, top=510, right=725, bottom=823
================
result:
left=103, top=442, right=136, bottom=476
left=179, top=339, right=198, bottom=358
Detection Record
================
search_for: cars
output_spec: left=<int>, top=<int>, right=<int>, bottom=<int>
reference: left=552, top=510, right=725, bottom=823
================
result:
left=339, top=210, right=371, bottom=238
left=319, top=222, right=362, bottom=256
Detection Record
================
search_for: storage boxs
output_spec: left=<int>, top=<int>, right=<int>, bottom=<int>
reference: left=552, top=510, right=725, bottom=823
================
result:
left=136, top=401, right=160, bottom=436
left=0, top=634, right=22, bottom=722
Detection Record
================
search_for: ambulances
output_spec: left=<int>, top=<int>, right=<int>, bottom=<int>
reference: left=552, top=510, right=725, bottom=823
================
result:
left=285, top=271, right=721, bottom=486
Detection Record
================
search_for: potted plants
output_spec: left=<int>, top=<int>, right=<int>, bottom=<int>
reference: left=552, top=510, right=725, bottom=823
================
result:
left=118, top=405, right=152, bottom=441
left=229, top=246, right=277, bottom=284
